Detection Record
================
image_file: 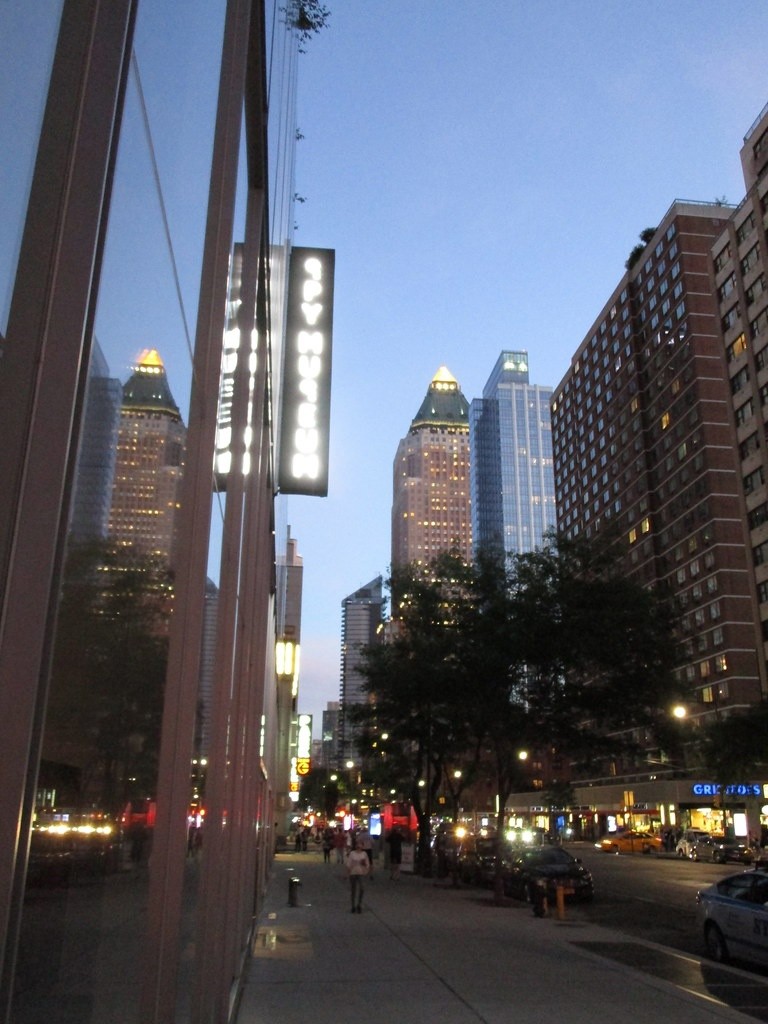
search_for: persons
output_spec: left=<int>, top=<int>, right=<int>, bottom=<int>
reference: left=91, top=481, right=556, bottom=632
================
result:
left=295, top=824, right=406, bottom=881
left=349, top=840, right=369, bottom=913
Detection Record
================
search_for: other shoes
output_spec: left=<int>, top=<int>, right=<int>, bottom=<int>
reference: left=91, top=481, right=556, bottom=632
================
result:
left=352, top=908, right=356, bottom=913
left=357, top=906, right=362, bottom=913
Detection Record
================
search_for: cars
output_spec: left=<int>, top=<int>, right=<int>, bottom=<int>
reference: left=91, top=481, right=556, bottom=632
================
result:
left=695, top=866, right=768, bottom=972
left=502, top=843, right=594, bottom=904
left=687, top=835, right=755, bottom=867
left=593, top=830, right=662, bottom=855
left=295, top=803, right=578, bottom=892
left=30, top=803, right=151, bottom=869
left=675, top=829, right=711, bottom=856
